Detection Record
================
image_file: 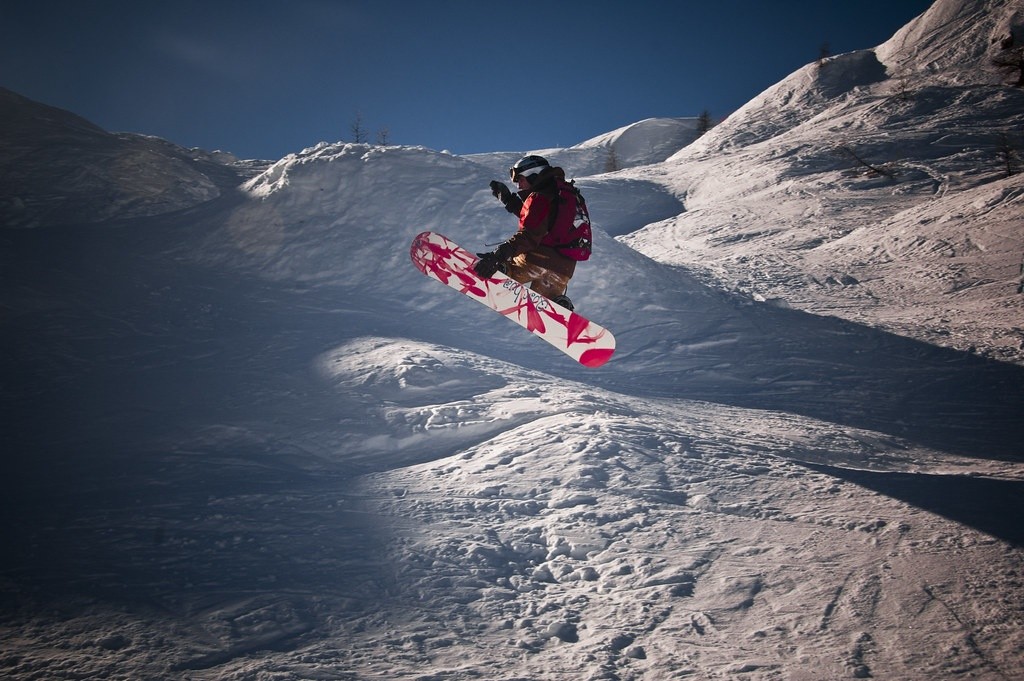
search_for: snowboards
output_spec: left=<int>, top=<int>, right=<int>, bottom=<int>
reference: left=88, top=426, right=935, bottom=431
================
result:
left=409, top=231, right=616, bottom=369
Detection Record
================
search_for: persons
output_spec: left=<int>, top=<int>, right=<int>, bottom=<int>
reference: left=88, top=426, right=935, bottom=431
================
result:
left=473, top=155, right=593, bottom=311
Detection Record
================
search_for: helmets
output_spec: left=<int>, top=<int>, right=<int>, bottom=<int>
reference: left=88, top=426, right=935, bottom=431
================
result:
left=509, top=154, right=551, bottom=184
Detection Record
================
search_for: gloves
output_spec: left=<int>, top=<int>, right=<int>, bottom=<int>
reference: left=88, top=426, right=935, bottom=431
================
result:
left=473, top=251, right=500, bottom=280
left=489, top=179, right=510, bottom=206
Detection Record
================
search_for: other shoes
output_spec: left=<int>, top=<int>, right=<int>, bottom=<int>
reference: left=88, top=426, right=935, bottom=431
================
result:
left=552, top=295, right=575, bottom=312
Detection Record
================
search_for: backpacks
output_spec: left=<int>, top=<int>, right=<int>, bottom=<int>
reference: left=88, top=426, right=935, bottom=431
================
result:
left=540, top=178, right=591, bottom=262
